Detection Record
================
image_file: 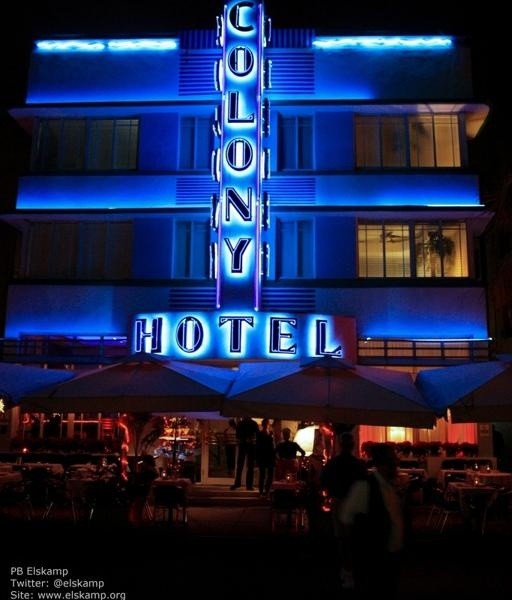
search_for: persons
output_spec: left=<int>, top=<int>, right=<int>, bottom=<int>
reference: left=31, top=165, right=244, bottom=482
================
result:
left=2, top=406, right=510, bottom=600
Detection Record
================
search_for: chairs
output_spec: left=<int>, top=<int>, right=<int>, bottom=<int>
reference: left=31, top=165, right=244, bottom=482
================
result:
left=265, top=453, right=512, bottom=582
left=0, top=460, right=191, bottom=544
